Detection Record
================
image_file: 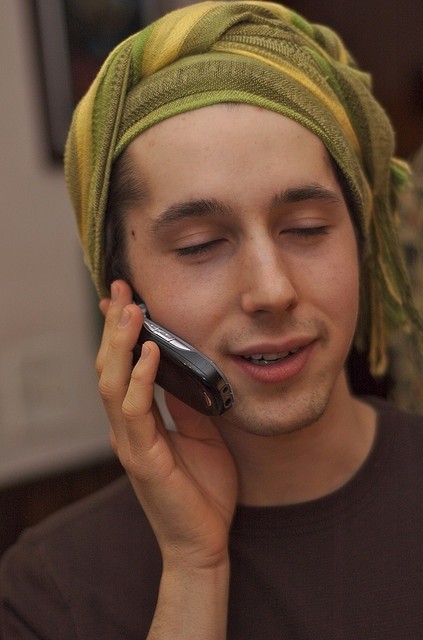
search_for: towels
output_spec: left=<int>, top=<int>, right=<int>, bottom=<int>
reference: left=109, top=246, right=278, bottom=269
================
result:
left=65, top=0, right=422, bottom=377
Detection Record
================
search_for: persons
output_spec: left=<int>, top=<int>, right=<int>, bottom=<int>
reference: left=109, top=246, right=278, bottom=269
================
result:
left=0, top=1, right=423, bottom=639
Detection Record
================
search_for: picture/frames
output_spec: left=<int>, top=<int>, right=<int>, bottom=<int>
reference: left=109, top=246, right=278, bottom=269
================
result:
left=32, top=1, right=162, bottom=161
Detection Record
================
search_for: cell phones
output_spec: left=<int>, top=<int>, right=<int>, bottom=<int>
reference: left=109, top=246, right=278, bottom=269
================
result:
left=133, top=304, right=235, bottom=417
